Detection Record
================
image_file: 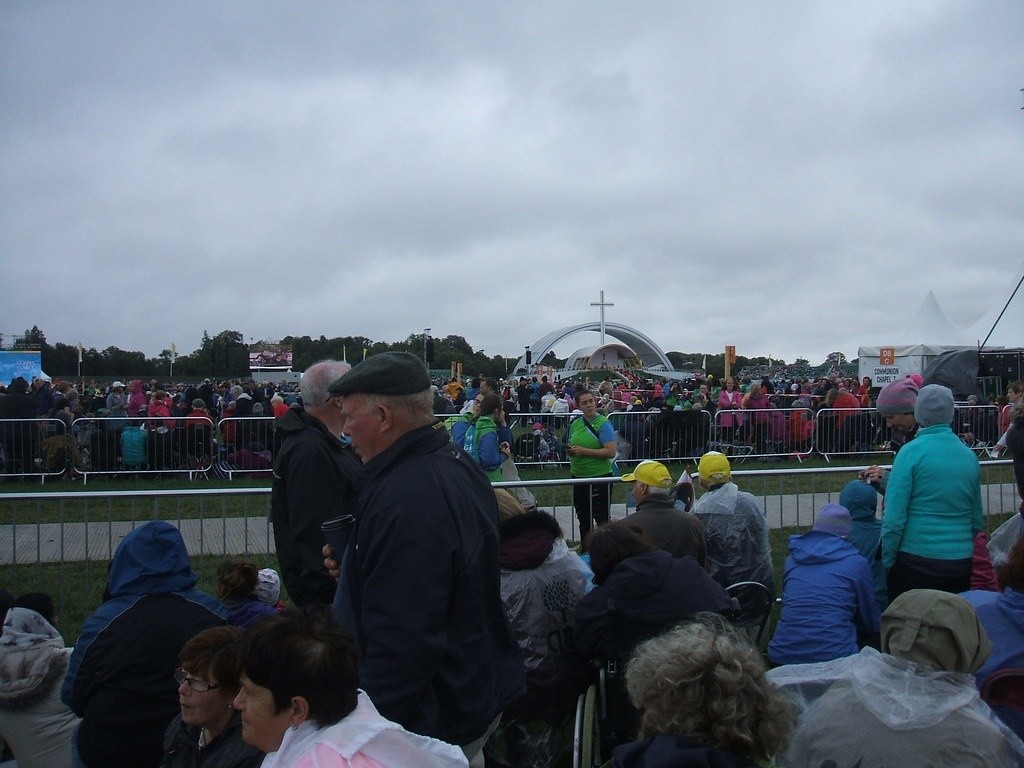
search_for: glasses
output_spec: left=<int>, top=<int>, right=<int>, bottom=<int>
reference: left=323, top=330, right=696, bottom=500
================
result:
left=473, top=398, right=483, bottom=405
left=172, top=668, right=237, bottom=694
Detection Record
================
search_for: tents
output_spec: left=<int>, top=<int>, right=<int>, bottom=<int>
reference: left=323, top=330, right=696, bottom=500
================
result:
left=858, top=345, right=1005, bottom=393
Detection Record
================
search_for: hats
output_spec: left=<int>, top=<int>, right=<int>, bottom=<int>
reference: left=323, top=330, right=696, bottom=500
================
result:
left=875, top=374, right=923, bottom=414
left=113, top=382, right=126, bottom=388
left=813, top=504, right=853, bottom=538
left=621, top=457, right=673, bottom=488
left=192, top=399, right=206, bottom=408
left=698, top=450, right=732, bottom=483
left=328, top=351, right=430, bottom=396
left=914, top=384, right=955, bottom=428
left=254, top=568, right=281, bottom=604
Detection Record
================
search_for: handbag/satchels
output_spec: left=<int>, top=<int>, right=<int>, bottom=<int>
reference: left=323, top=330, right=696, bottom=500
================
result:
left=986, top=514, right=1024, bottom=570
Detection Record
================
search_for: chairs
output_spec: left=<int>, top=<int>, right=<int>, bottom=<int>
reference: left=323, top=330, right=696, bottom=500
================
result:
left=513, top=433, right=541, bottom=469
left=980, top=668, right=1024, bottom=713
left=707, top=439, right=784, bottom=465
left=724, top=581, right=773, bottom=648
left=572, top=663, right=608, bottom=768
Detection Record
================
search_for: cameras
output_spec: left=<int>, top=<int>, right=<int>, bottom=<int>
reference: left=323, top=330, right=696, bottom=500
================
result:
left=859, top=470, right=872, bottom=485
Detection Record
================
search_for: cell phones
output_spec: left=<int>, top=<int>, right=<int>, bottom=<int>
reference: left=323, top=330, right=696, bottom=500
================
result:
left=566, top=446, right=573, bottom=449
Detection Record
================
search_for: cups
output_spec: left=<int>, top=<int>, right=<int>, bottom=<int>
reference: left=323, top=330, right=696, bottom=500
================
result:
left=322, top=514, right=355, bottom=567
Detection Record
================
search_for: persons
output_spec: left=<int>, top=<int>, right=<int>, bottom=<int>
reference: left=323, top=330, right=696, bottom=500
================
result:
left=254, top=354, right=290, bottom=366
left=491, top=452, right=775, bottom=742
left=652, top=374, right=775, bottom=461
left=763, top=374, right=1024, bottom=767
left=269, top=357, right=364, bottom=607
left=608, top=610, right=804, bottom=768
left=527, top=374, right=650, bottom=465
left=0, top=520, right=287, bottom=768
left=233, top=602, right=472, bottom=768
left=0, top=375, right=180, bottom=483
left=173, top=376, right=305, bottom=478
left=563, top=389, right=616, bottom=553
left=429, top=373, right=537, bottom=512
left=319, top=352, right=525, bottom=768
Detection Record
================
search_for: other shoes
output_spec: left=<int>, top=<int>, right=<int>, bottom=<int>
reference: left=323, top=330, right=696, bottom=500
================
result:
left=571, top=544, right=583, bottom=554
